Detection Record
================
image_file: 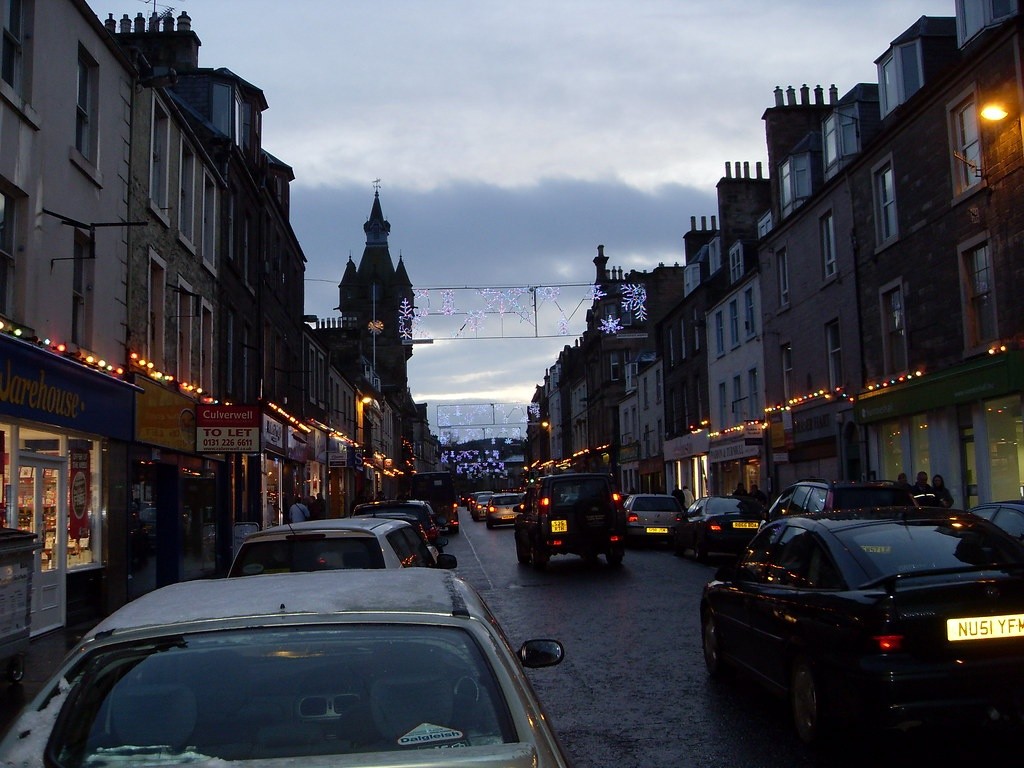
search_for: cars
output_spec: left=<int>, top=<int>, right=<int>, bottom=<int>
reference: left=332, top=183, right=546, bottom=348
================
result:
left=674, top=495, right=767, bottom=564
left=1, top=568, right=569, bottom=768
left=370, top=512, right=448, bottom=566
left=700, top=502, right=1024, bottom=751
left=466, top=490, right=523, bottom=529
left=623, top=493, right=683, bottom=545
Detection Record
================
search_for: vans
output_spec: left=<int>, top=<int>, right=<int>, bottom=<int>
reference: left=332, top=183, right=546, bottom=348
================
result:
left=227, top=515, right=458, bottom=572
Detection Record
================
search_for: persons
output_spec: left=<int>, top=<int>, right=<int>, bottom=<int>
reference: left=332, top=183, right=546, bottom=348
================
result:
left=886, top=472, right=954, bottom=508
left=732, top=482, right=766, bottom=505
left=289, top=493, right=327, bottom=523
left=128, top=467, right=182, bottom=602
left=397, top=489, right=411, bottom=503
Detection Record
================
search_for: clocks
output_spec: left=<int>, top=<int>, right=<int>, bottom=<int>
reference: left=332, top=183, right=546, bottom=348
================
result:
left=367, top=319, right=384, bottom=335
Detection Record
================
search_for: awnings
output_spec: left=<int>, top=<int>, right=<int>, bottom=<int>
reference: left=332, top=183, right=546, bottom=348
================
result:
left=503, top=455, right=525, bottom=463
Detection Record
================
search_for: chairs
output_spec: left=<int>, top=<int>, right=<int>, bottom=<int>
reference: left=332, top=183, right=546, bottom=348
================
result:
left=111, top=683, right=198, bottom=749
left=358, top=674, right=465, bottom=747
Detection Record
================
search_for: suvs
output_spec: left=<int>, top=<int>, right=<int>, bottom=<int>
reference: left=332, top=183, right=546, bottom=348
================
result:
left=512, top=473, right=629, bottom=569
left=354, top=498, right=449, bottom=554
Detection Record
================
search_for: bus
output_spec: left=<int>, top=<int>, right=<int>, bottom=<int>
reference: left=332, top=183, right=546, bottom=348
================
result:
left=737, top=478, right=922, bottom=583
left=407, top=470, right=460, bottom=535
